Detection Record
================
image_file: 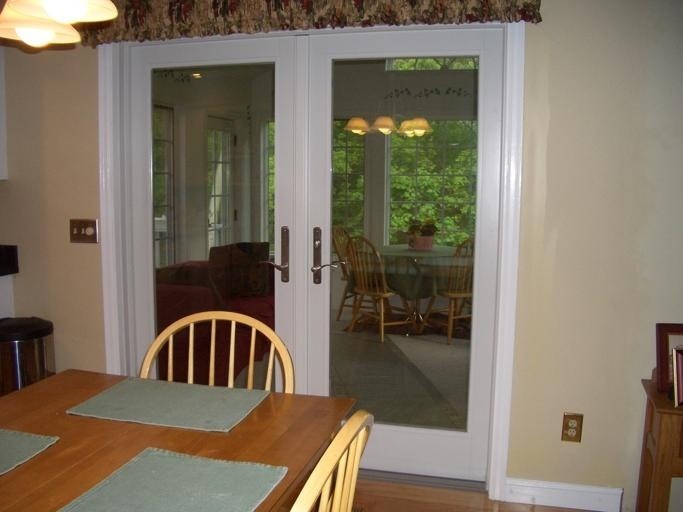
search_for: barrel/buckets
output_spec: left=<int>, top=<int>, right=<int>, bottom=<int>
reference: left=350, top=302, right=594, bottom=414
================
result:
left=412, top=234, right=435, bottom=249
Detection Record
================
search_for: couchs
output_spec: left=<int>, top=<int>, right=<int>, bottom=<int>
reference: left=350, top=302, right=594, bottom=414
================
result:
left=153, top=261, right=272, bottom=386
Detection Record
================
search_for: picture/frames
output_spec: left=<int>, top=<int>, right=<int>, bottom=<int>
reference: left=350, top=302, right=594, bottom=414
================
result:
left=672, top=348, right=683, bottom=408
left=655, top=322, right=683, bottom=391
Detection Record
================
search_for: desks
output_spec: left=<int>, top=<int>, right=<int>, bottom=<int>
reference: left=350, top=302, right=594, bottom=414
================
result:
left=636, top=378, right=683, bottom=512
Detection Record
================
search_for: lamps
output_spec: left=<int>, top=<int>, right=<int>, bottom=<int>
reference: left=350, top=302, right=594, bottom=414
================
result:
left=0, top=0, right=118, bottom=49
left=342, top=57, right=434, bottom=138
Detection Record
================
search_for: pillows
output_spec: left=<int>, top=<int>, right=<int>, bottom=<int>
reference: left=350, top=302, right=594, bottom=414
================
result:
left=157, top=264, right=226, bottom=309
left=209, top=242, right=270, bottom=299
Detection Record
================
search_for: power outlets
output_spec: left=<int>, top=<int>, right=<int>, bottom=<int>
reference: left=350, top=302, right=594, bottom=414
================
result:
left=561, top=412, right=583, bottom=442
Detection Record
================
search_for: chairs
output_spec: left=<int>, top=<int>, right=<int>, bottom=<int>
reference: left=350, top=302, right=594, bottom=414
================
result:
left=138, top=311, right=295, bottom=395
left=292, top=406, right=375, bottom=511
left=333, top=227, right=473, bottom=346
